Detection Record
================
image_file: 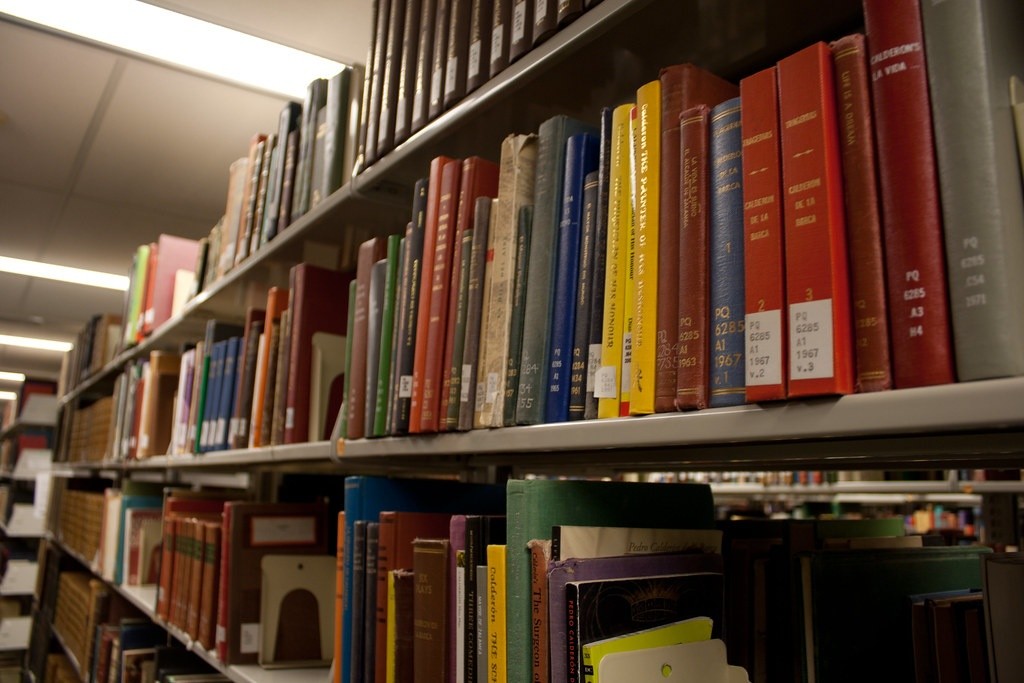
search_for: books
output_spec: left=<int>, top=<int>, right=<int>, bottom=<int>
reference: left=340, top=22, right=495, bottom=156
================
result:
left=0, top=1, right=1024, bottom=683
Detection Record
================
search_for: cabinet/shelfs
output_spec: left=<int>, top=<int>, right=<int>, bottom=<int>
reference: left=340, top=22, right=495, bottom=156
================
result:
left=19, top=0, right=1024, bottom=683
left=0, top=372, right=63, bottom=667
